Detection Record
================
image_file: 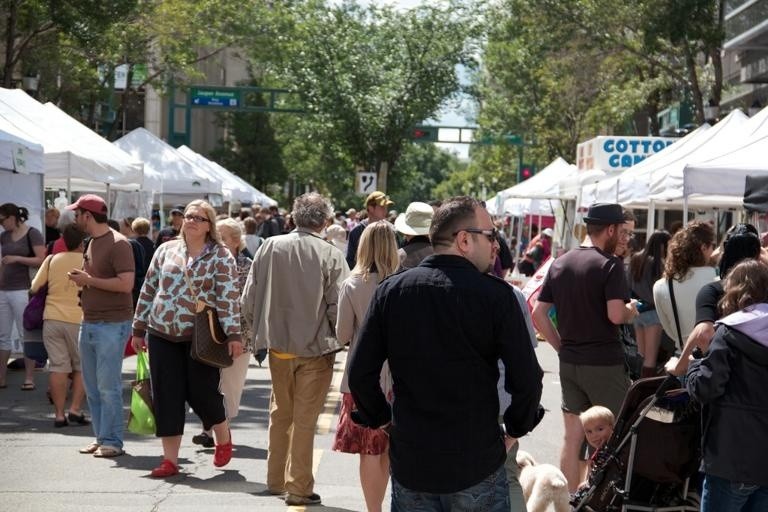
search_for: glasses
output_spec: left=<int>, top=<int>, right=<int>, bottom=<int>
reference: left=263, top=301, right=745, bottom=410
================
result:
left=185, top=215, right=210, bottom=222
left=453, top=228, right=495, bottom=240
left=0, top=216, right=9, bottom=223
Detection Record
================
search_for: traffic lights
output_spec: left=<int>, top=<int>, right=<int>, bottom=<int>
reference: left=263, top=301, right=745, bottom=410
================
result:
left=412, top=127, right=437, bottom=143
left=522, top=165, right=531, bottom=180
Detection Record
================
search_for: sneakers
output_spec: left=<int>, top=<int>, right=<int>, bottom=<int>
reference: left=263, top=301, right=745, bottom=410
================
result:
left=55, top=413, right=89, bottom=428
left=285, top=493, right=321, bottom=505
left=154, top=431, right=231, bottom=477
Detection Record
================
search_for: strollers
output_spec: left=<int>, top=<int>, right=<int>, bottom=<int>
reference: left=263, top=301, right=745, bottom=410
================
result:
left=571, top=375, right=700, bottom=511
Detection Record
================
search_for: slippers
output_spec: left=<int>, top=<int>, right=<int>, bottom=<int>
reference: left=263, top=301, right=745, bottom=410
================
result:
left=81, top=441, right=125, bottom=457
left=21, top=383, right=35, bottom=390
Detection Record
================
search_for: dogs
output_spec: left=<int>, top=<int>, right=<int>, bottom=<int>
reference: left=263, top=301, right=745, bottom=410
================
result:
left=515, top=449, right=571, bottom=512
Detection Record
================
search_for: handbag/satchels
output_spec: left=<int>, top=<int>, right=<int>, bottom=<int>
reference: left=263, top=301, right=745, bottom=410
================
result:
left=127, top=350, right=156, bottom=434
left=23, top=285, right=47, bottom=331
left=190, top=300, right=233, bottom=368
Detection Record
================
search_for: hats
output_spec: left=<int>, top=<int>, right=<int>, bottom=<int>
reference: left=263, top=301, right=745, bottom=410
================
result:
left=394, top=202, right=435, bottom=235
left=65, top=194, right=108, bottom=215
left=367, top=191, right=394, bottom=207
left=583, top=203, right=625, bottom=224
left=170, top=205, right=185, bottom=214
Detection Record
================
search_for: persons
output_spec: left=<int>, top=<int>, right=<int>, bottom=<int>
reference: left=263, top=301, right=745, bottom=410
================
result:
left=348, top=195, right=544, bottom=512
left=335, top=220, right=401, bottom=512
left=532, top=204, right=637, bottom=494
left=497, top=290, right=537, bottom=512
left=0, top=193, right=767, bottom=479
left=686, top=259, right=768, bottom=512
left=241, top=192, right=349, bottom=506
